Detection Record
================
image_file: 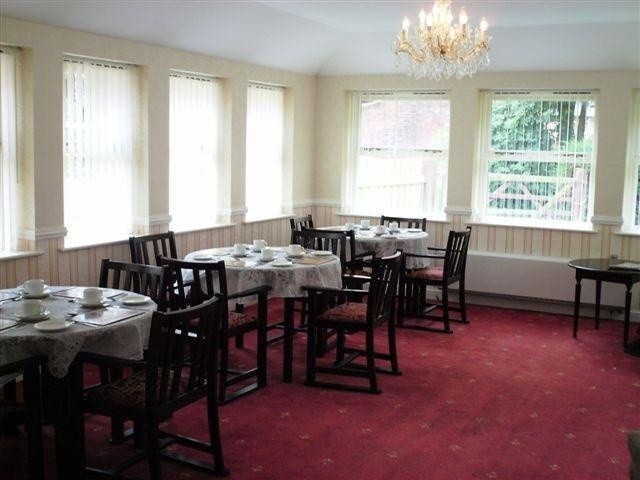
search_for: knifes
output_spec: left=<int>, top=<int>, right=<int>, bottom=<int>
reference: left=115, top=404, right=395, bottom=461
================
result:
left=106, top=292, right=127, bottom=301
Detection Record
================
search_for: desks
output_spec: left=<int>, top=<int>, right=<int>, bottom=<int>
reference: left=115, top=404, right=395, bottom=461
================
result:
left=566, top=256, right=640, bottom=351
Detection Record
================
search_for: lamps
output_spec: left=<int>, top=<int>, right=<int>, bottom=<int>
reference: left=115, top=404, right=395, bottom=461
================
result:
left=384, top=0, right=495, bottom=84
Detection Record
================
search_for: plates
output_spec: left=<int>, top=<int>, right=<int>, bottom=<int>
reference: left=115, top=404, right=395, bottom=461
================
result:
left=118, top=295, right=151, bottom=305
left=271, top=262, right=293, bottom=266
left=608, top=262, right=640, bottom=271
left=16, top=310, right=50, bottom=321
left=77, top=299, right=106, bottom=307
left=193, top=254, right=213, bottom=259
left=21, top=291, right=50, bottom=300
left=342, top=219, right=423, bottom=238
left=34, top=320, right=73, bottom=331
left=311, top=250, right=333, bottom=255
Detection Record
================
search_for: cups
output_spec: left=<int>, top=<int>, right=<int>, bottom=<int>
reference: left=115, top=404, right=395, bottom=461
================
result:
left=233, top=237, right=306, bottom=262
left=22, top=299, right=46, bottom=317
left=25, top=280, right=44, bottom=295
left=83, top=288, right=103, bottom=303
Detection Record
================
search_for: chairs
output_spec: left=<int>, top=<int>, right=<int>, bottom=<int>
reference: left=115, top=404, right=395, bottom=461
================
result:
left=0, top=213, right=471, bottom=478
